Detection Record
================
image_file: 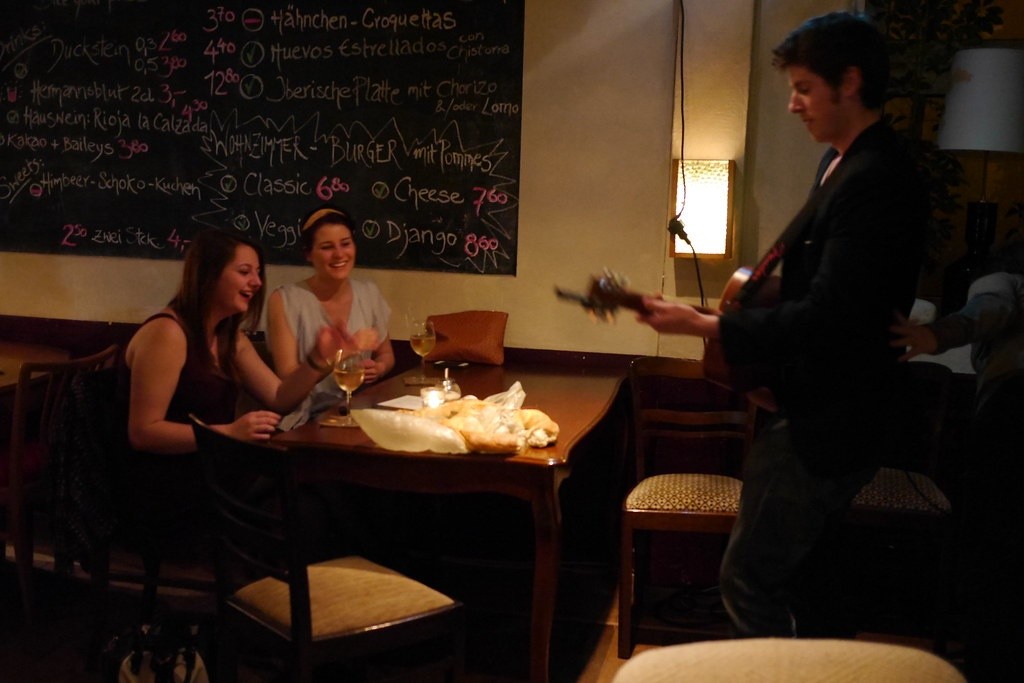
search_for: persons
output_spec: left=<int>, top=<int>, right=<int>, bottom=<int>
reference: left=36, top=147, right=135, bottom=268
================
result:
left=639, top=10, right=925, bottom=638
left=266, top=205, right=396, bottom=414
left=94, top=224, right=362, bottom=578
left=889, top=267, right=1024, bottom=683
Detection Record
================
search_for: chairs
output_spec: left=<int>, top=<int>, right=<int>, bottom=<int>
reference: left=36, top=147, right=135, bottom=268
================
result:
left=0, top=343, right=973, bottom=683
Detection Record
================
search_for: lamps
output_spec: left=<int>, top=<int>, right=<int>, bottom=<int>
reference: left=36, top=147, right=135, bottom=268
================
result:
left=668, top=159, right=736, bottom=259
left=932, top=47, right=1024, bottom=249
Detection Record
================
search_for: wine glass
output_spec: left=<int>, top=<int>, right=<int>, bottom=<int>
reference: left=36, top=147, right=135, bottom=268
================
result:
left=410, top=320, right=436, bottom=384
left=332, top=349, right=365, bottom=427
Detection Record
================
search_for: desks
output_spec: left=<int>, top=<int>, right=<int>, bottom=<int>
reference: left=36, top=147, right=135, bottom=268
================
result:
left=0, top=341, right=71, bottom=450
left=268, top=359, right=628, bottom=683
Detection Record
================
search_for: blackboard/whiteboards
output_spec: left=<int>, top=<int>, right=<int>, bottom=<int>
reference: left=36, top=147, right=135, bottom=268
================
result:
left=0, top=0, right=525, bottom=276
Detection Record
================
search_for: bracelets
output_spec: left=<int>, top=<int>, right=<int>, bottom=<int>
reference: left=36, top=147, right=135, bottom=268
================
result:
left=305, top=354, right=328, bottom=373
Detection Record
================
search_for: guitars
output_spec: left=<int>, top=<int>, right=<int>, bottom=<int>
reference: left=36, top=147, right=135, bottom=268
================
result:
left=551, top=267, right=783, bottom=418
